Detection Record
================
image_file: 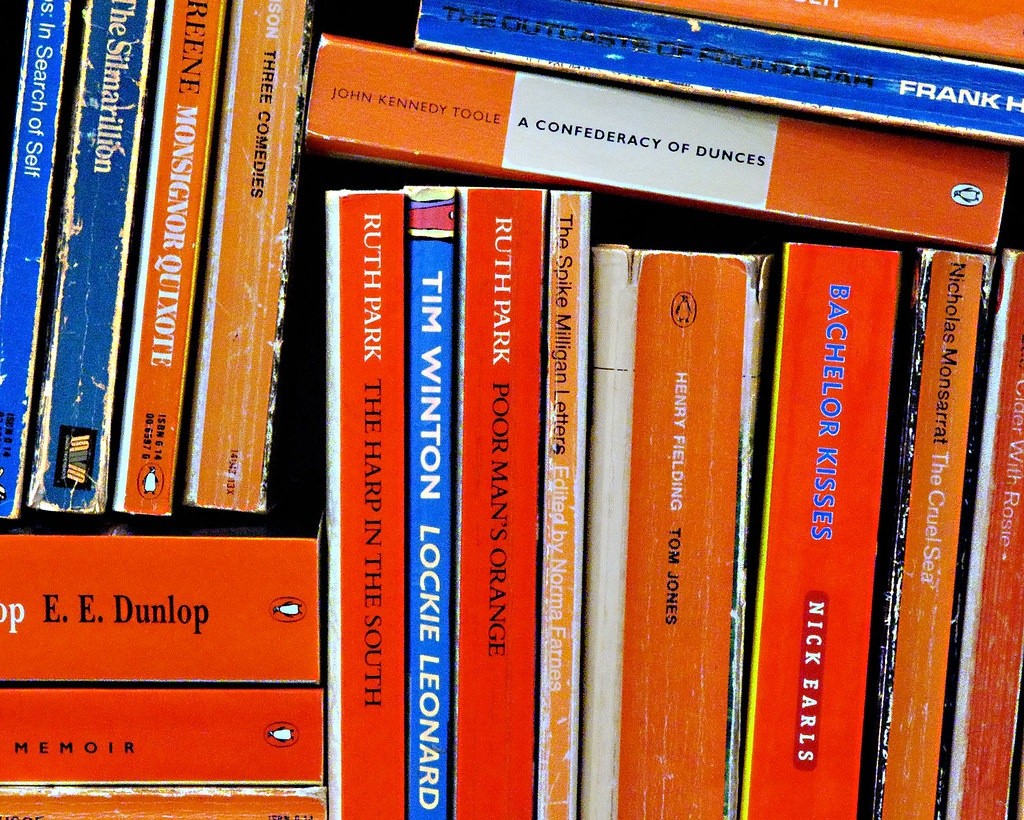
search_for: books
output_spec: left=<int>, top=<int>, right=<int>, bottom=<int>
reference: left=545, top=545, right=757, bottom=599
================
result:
left=0, top=512, right=324, bottom=683
left=583, top=240, right=772, bottom=820
left=305, top=34, right=1010, bottom=251
left=0, top=0, right=1024, bottom=820
